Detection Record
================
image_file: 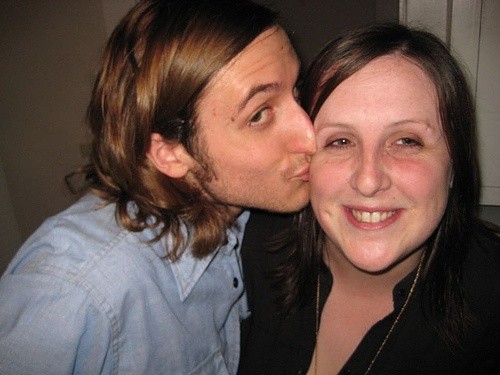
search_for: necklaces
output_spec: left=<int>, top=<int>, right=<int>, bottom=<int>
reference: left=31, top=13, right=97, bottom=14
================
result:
left=314, top=234, right=425, bottom=374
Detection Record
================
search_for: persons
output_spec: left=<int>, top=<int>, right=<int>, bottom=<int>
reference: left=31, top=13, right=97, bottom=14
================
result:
left=0, top=0, right=316, bottom=375
left=236, top=22, right=500, bottom=375
